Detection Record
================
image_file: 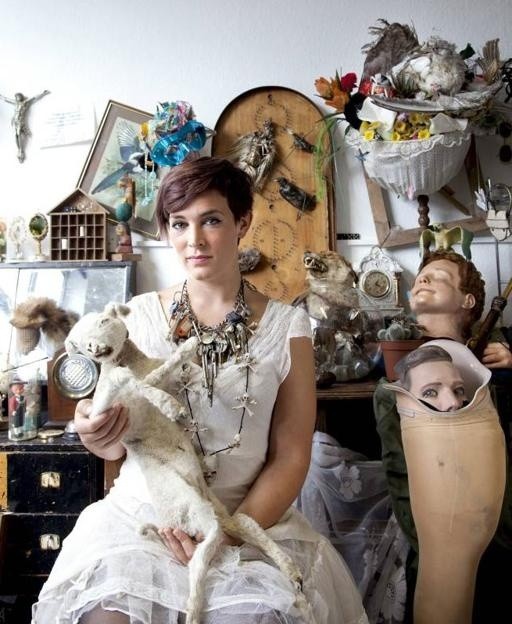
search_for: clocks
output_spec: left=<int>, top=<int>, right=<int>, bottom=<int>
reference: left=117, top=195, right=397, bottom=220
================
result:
left=354, top=246, right=405, bottom=322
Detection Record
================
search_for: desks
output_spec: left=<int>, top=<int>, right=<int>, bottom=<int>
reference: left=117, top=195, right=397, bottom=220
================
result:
left=314, top=380, right=496, bottom=451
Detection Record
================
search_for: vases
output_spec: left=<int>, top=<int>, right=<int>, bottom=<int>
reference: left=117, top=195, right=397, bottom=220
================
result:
left=360, top=132, right=471, bottom=202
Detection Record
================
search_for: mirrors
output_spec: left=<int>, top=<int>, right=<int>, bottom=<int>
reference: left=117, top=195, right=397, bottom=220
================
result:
left=0, top=258, right=134, bottom=385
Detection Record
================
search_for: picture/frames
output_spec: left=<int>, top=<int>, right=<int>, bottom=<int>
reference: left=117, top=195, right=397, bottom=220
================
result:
left=363, top=132, right=489, bottom=248
left=75, top=99, right=194, bottom=241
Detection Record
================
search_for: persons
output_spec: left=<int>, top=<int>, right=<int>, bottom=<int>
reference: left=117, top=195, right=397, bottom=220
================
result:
left=0, top=88, right=50, bottom=164
left=30, top=157, right=370, bottom=623
left=9, top=378, right=28, bottom=437
left=407, top=251, right=512, bottom=368
left=392, top=345, right=467, bottom=413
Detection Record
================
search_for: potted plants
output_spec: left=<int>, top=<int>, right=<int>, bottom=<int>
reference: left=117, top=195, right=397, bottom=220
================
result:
left=377, top=316, right=425, bottom=381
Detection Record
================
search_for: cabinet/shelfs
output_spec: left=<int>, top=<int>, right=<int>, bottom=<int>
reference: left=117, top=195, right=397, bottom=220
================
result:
left=0, top=426, right=122, bottom=624
left=47, top=187, right=110, bottom=262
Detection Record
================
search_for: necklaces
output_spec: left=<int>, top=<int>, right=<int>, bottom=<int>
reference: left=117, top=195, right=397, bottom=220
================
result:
left=167, top=271, right=257, bottom=488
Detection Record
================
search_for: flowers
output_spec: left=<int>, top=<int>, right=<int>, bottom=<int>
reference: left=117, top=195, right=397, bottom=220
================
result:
left=310, top=72, right=435, bottom=202
